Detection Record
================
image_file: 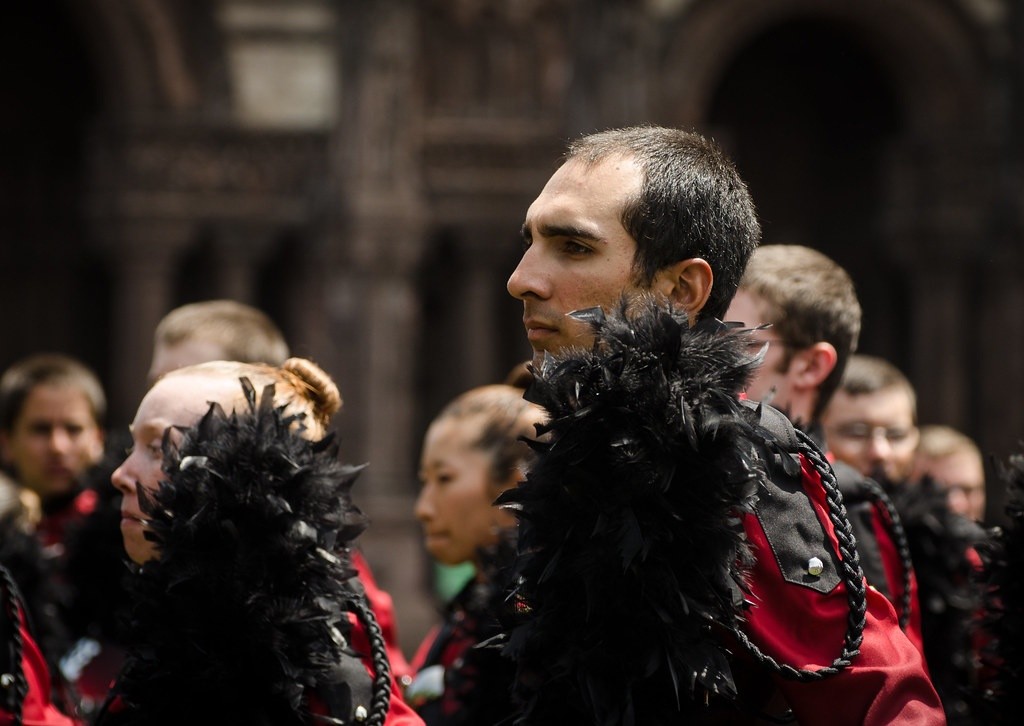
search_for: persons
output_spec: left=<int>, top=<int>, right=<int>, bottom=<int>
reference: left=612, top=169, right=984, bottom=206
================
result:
left=405, top=126, right=1024, bottom=726
left=0, top=299, right=425, bottom=726
